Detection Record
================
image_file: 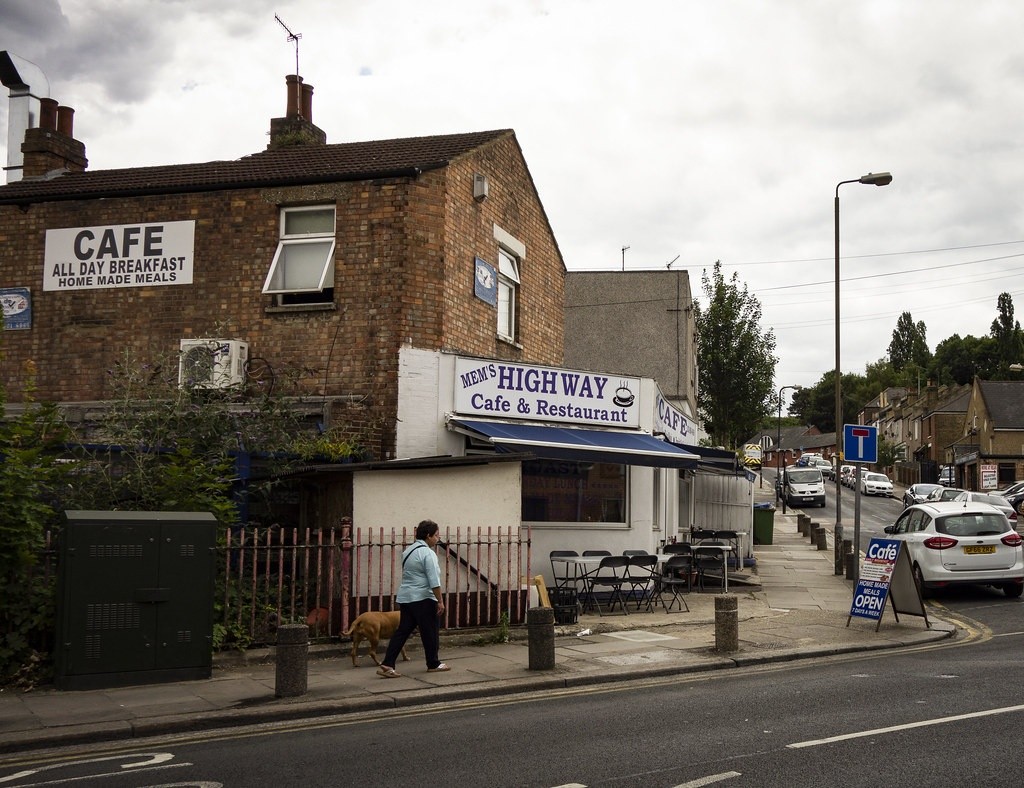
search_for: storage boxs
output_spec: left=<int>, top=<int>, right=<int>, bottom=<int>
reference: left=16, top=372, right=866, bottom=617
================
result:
left=539, top=587, right=577, bottom=627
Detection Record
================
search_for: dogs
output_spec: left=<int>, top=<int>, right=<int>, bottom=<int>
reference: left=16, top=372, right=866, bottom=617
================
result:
left=340, top=610, right=410, bottom=669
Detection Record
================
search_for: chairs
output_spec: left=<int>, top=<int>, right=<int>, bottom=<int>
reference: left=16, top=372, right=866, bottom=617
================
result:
left=581, top=556, right=630, bottom=617
left=646, top=555, right=692, bottom=614
left=623, top=550, right=665, bottom=609
left=663, top=529, right=739, bottom=595
left=582, top=551, right=626, bottom=615
left=550, top=551, right=592, bottom=616
left=609, top=555, right=658, bottom=613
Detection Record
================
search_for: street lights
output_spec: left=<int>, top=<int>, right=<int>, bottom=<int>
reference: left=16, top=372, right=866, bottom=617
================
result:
left=776, top=385, right=802, bottom=507
left=833, top=169, right=894, bottom=598
left=760, top=395, right=779, bottom=489
left=776, top=447, right=793, bottom=513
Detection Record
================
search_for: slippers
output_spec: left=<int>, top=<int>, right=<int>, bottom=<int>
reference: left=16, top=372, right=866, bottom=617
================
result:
left=376, top=669, right=401, bottom=677
left=427, top=664, right=451, bottom=671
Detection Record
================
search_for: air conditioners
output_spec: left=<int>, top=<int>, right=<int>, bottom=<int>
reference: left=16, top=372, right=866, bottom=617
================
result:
left=178, top=338, right=248, bottom=391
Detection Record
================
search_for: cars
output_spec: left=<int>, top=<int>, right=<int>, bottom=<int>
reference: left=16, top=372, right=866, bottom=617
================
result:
left=988, top=480, right=1024, bottom=516
left=774, top=452, right=870, bottom=509
left=903, top=483, right=948, bottom=510
left=949, top=490, right=1018, bottom=531
left=922, top=486, right=966, bottom=503
left=883, top=500, right=1024, bottom=601
left=860, top=471, right=894, bottom=498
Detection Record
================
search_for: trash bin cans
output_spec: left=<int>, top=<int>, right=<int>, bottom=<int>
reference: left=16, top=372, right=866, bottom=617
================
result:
left=753, top=503, right=776, bottom=545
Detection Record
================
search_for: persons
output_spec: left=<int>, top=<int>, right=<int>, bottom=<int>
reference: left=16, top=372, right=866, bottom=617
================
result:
left=376, top=519, right=451, bottom=678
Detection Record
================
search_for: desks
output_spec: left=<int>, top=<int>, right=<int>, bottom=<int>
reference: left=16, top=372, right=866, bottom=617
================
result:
left=551, top=555, right=635, bottom=613
left=658, top=546, right=732, bottom=593
left=684, top=530, right=747, bottom=571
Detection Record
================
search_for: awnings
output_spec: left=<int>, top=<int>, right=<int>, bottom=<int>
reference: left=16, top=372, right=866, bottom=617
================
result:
left=448, top=418, right=738, bottom=476
